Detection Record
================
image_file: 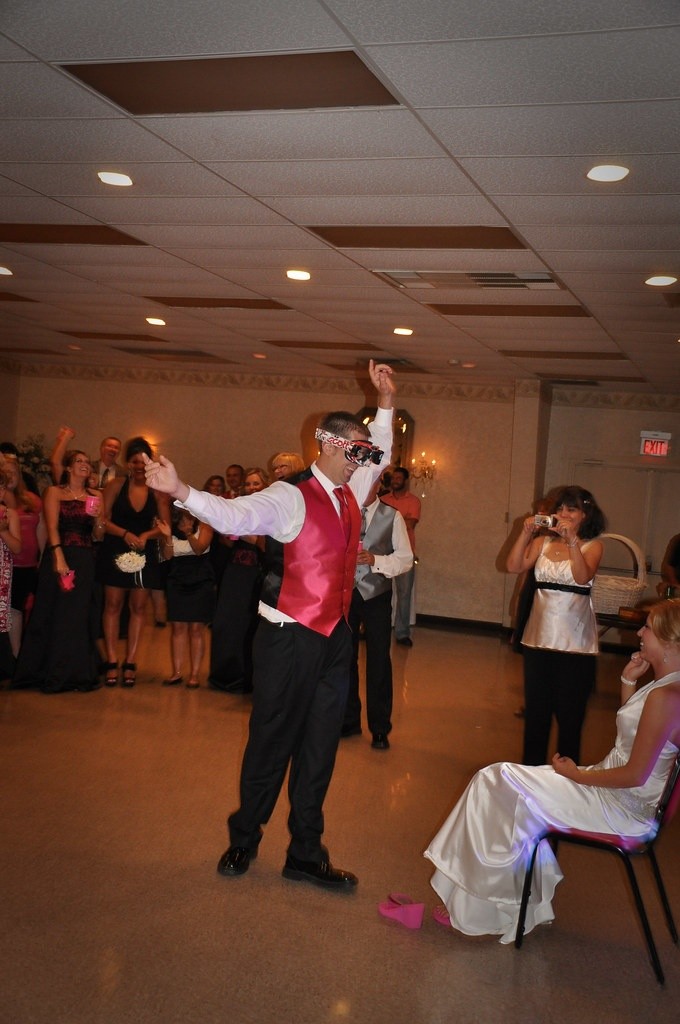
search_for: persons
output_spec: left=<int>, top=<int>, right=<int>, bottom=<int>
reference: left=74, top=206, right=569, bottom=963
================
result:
left=0, top=425, right=306, bottom=694
left=340, top=476, right=414, bottom=748
left=665, top=537, right=680, bottom=598
left=505, top=484, right=608, bottom=765
left=381, top=466, right=420, bottom=647
left=514, top=498, right=547, bottom=718
left=143, top=360, right=397, bottom=889
left=423, top=598, right=680, bottom=945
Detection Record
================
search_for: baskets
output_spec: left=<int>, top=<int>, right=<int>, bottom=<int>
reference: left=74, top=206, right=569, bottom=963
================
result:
left=588, top=533, right=650, bottom=614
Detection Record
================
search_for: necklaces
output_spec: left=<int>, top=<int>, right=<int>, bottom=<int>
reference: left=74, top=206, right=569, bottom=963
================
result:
left=67, top=484, right=85, bottom=499
left=555, top=552, right=559, bottom=556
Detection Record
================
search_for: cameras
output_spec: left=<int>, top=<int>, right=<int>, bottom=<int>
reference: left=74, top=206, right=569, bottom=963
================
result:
left=534, top=514, right=554, bottom=529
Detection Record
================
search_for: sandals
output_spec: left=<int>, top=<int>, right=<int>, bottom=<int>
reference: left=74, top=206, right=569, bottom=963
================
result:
left=121, top=661, right=137, bottom=687
left=103, top=661, right=119, bottom=686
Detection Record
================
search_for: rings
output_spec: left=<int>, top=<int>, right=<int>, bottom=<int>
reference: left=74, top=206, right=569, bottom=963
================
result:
left=130, top=543, right=134, bottom=546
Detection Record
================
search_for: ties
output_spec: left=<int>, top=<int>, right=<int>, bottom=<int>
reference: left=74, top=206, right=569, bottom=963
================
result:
left=333, top=488, right=352, bottom=546
left=359, top=508, right=368, bottom=542
left=100, top=468, right=110, bottom=488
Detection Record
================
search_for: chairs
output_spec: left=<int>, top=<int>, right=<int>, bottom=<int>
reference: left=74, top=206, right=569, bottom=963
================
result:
left=514, top=755, right=680, bottom=985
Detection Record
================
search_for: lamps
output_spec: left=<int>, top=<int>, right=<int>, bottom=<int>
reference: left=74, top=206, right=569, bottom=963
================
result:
left=409, top=450, right=436, bottom=499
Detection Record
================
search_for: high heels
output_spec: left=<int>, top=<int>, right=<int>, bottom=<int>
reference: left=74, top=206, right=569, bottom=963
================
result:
left=432, top=906, right=452, bottom=927
left=378, top=891, right=425, bottom=928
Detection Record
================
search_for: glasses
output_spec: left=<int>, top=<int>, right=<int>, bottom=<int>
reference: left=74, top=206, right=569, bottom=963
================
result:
left=330, top=436, right=384, bottom=467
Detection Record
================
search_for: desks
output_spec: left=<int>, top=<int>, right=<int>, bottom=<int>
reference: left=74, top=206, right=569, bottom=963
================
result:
left=595, top=610, right=648, bottom=657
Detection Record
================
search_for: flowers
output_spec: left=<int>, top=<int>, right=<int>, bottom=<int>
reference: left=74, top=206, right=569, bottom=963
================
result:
left=114, top=552, right=146, bottom=572
left=17, top=432, right=52, bottom=482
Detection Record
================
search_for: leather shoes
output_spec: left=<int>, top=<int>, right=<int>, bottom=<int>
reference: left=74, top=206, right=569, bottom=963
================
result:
left=338, top=726, right=362, bottom=737
left=282, top=852, right=358, bottom=891
left=372, top=732, right=390, bottom=748
left=216, top=841, right=258, bottom=877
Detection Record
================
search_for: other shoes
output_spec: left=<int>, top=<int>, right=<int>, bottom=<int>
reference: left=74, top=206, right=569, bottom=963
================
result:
left=513, top=705, right=526, bottom=718
left=395, top=635, right=412, bottom=647
left=186, top=670, right=201, bottom=688
left=163, top=670, right=183, bottom=685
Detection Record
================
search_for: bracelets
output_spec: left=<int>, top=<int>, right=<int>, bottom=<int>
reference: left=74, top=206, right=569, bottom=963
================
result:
left=621, top=676, right=637, bottom=686
left=165, top=542, right=174, bottom=547
left=97, top=522, right=106, bottom=528
left=122, top=531, right=128, bottom=539
left=51, top=544, right=61, bottom=551
left=567, top=536, right=578, bottom=547
